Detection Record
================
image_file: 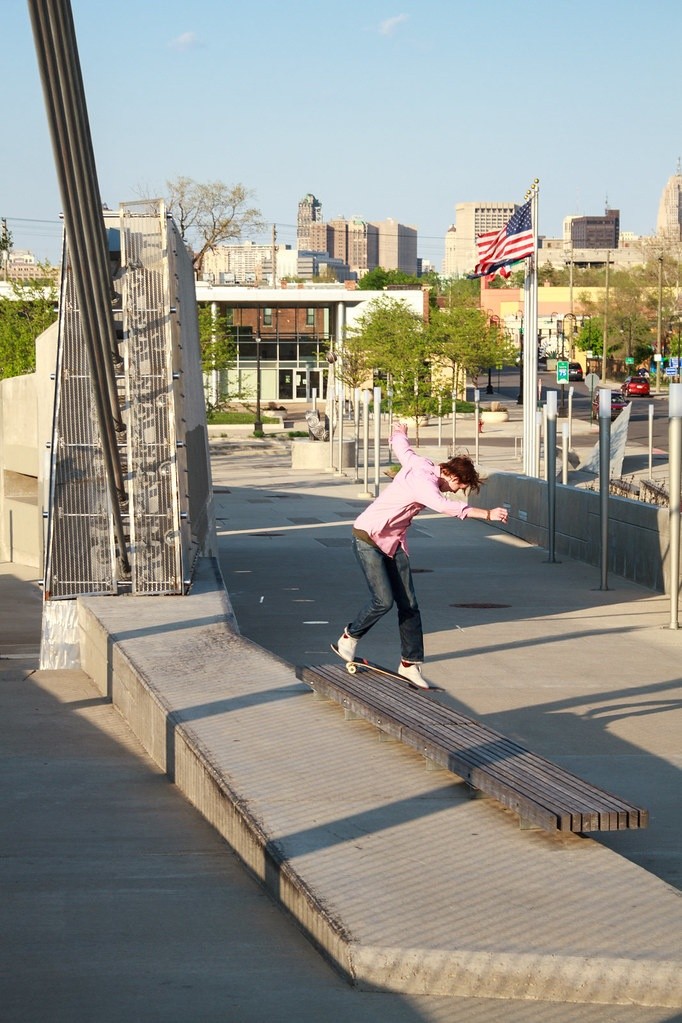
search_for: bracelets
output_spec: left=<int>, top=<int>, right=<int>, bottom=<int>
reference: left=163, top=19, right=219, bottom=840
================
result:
left=487, top=510, right=490, bottom=521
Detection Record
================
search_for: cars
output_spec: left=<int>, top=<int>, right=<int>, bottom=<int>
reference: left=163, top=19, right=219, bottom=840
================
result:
left=638, top=369, right=648, bottom=375
left=620, top=376, right=650, bottom=397
left=591, top=390, right=630, bottom=422
left=568, top=362, right=583, bottom=381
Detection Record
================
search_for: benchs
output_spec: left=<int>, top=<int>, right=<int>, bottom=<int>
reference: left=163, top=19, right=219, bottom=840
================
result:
left=300, top=656, right=650, bottom=841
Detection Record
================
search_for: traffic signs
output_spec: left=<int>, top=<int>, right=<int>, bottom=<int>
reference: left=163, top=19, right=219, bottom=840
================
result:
left=625, top=357, right=634, bottom=365
left=557, top=361, right=569, bottom=385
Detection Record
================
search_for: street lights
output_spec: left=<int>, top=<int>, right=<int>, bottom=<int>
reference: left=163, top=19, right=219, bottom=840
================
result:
left=619, top=316, right=632, bottom=376
left=580, top=314, right=591, bottom=371
left=253, top=329, right=263, bottom=432
left=557, top=312, right=579, bottom=419
left=515, top=310, right=523, bottom=404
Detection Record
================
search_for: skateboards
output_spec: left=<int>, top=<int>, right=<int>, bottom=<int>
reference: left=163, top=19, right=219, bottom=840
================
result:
left=329, top=643, right=445, bottom=694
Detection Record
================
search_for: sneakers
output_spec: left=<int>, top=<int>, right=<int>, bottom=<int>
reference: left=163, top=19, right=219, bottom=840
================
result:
left=397, top=660, right=428, bottom=689
left=337, top=632, right=357, bottom=662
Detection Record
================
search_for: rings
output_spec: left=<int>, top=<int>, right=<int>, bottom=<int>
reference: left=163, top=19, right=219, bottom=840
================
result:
left=501, top=518, right=503, bottom=522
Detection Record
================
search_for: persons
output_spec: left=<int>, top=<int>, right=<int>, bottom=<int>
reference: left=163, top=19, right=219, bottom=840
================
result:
left=338, top=421, right=510, bottom=688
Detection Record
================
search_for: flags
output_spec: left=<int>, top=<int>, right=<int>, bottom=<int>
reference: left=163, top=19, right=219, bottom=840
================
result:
left=465, top=200, right=535, bottom=280
